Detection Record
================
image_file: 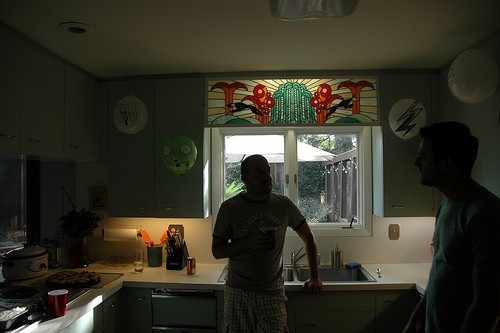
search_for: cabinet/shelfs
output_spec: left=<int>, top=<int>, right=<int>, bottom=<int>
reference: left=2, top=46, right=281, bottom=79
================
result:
left=372, top=35, right=500, bottom=218
left=0, top=25, right=213, bottom=218
left=57, top=286, right=427, bottom=333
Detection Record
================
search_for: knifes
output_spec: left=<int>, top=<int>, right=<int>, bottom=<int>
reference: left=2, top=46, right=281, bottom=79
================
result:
left=164, top=230, right=182, bottom=256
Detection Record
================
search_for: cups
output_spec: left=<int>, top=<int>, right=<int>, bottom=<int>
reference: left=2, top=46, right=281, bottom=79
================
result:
left=48, top=289, right=69, bottom=316
left=146, top=244, right=163, bottom=267
left=259, top=227, right=278, bottom=250
left=133, top=248, right=144, bottom=272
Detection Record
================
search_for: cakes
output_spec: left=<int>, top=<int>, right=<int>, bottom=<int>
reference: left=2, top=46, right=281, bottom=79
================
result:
left=45, top=270, right=101, bottom=286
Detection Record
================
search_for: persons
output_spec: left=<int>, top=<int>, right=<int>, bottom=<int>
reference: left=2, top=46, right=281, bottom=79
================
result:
left=211, top=155, right=323, bottom=333
left=402, top=121, right=500, bottom=333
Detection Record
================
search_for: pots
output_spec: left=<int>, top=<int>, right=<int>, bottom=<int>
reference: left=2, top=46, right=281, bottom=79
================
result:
left=0, top=242, right=48, bottom=280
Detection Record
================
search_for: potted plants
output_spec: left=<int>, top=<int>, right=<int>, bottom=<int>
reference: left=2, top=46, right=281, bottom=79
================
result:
left=56, top=207, right=101, bottom=266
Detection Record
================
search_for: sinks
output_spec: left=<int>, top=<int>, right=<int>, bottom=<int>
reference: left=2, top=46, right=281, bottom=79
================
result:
left=297, top=265, right=377, bottom=283
left=217, top=264, right=294, bottom=283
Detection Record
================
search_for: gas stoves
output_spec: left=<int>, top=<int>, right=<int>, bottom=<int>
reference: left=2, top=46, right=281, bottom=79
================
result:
left=0, top=281, right=91, bottom=333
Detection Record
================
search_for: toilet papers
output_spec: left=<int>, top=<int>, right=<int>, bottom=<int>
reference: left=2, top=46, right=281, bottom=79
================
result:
left=103, top=228, right=139, bottom=241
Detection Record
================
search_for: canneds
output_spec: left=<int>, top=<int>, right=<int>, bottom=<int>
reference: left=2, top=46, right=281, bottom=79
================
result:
left=186, top=256, right=196, bottom=275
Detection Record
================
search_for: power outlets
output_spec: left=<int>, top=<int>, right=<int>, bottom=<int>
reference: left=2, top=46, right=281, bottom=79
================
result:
left=168, top=224, right=184, bottom=240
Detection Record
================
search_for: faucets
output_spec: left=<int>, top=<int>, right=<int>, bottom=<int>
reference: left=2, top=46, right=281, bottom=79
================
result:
left=282, top=247, right=308, bottom=269
left=316, top=250, right=321, bottom=268
